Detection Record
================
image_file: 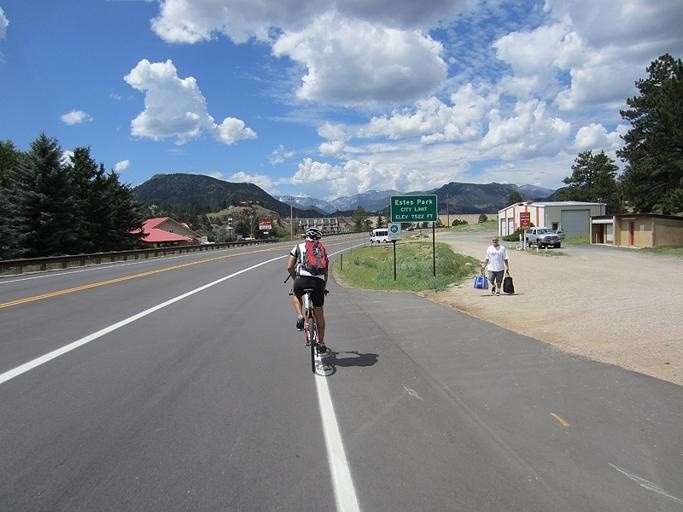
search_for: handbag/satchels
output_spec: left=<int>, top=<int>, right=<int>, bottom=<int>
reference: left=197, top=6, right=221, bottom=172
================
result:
left=504, top=276, right=514, bottom=293
left=474, top=276, right=488, bottom=289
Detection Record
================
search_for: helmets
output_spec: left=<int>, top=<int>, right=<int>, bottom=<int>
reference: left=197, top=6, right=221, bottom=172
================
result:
left=303, top=228, right=323, bottom=240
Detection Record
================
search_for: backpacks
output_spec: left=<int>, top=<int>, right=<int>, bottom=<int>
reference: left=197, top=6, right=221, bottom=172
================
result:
left=303, top=241, right=328, bottom=275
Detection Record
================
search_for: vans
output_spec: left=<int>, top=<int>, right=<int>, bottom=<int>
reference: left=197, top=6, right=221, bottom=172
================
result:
left=370, top=227, right=396, bottom=244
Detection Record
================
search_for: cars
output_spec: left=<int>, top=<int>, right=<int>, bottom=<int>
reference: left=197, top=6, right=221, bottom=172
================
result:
left=524, top=226, right=563, bottom=247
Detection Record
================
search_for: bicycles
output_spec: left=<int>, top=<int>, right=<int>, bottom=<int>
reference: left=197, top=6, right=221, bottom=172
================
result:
left=285, top=286, right=329, bottom=375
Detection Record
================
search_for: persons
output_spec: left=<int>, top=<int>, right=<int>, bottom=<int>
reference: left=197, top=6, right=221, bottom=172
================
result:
left=481, top=236, right=509, bottom=296
left=287, top=229, right=329, bottom=352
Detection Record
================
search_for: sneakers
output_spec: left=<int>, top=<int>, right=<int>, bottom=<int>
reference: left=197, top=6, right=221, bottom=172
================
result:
left=296, top=317, right=304, bottom=329
left=491, top=287, right=500, bottom=296
left=316, top=342, right=328, bottom=352
